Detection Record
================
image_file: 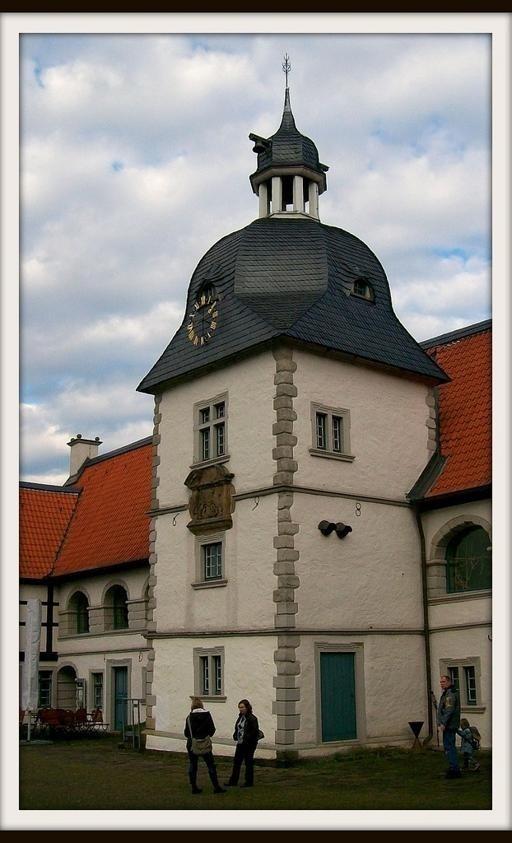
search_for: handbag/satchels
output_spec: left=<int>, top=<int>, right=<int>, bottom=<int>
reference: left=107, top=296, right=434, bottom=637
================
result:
left=191, top=736, right=212, bottom=756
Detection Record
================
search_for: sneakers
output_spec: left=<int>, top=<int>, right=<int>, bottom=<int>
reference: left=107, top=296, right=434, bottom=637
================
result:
left=192, top=788, right=203, bottom=794
left=240, top=782, right=254, bottom=788
left=213, top=788, right=228, bottom=794
left=443, top=771, right=465, bottom=779
left=223, top=782, right=238, bottom=786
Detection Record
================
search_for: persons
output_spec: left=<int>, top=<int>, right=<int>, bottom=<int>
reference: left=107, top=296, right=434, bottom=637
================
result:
left=221, top=698, right=259, bottom=787
left=434, top=673, right=461, bottom=780
left=184, top=698, right=225, bottom=795
left=456, top=717, right=480, bottom=770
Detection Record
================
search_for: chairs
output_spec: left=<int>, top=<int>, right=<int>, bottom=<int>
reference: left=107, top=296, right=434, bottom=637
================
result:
left=31, top=706, right=110, bottom=737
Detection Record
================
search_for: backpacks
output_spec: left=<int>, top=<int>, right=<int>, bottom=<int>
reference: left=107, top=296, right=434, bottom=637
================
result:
left=465, top=726, right=481, bottom=751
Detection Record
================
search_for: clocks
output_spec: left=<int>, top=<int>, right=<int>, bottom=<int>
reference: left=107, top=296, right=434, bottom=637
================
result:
left=186, top=296, right=219, bottom=347
